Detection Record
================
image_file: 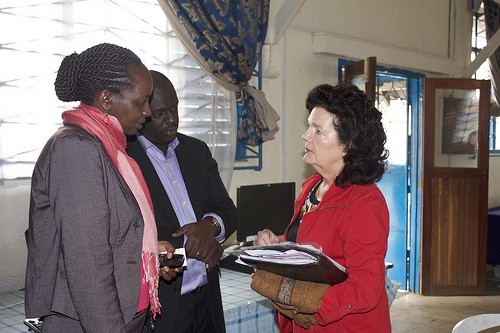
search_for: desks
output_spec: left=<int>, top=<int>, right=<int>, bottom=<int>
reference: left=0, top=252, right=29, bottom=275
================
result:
left=0, top=267, right=279, bottom=333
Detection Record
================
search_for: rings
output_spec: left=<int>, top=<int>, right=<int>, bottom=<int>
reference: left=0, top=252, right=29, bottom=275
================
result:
left=196, top=252, right=202, bottom=256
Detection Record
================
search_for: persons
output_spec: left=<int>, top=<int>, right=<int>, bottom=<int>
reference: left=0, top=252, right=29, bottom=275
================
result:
left=124, top=69, right=241, bottom=333
left=25, top=42, right=159, bottom=333
left=249, top=82, right=392, bottom=333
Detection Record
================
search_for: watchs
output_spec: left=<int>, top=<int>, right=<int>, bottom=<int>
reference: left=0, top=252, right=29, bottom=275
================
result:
left=204, top=215, right=221, bottom=236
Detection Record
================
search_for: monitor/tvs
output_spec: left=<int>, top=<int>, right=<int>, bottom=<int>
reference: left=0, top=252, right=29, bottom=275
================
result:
left=236, top=182, right=295, bottom=242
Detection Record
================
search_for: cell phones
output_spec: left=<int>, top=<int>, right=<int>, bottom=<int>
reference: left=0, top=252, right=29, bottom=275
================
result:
left=158, top=254, right=184, bottom=268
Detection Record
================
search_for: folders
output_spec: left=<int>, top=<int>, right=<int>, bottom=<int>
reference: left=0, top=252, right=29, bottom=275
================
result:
left=239, top=246, right=347, bottom=285
